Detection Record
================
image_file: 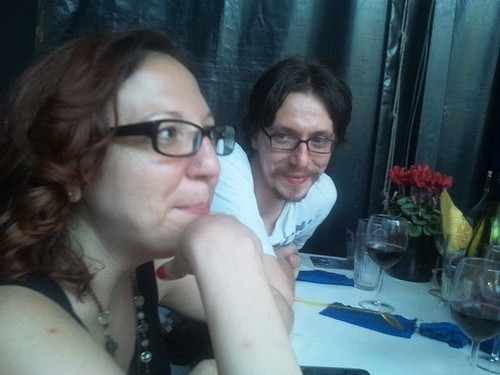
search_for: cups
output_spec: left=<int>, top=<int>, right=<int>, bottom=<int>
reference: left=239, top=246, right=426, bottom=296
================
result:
left=352, top=218, right=380, bottom=291
left=440, top=231, right=470, bottom=305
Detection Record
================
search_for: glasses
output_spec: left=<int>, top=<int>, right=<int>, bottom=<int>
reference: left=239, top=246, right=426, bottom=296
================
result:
left=258, top=124, right=337, bottom=154
left=108, top=119, right=237, bottom=157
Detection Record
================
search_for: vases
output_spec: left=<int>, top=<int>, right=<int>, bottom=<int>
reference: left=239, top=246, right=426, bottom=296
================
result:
left=379, top=222, right=437, bottom=283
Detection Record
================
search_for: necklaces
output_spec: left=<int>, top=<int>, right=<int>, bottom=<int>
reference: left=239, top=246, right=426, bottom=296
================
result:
left=89, top=283, right=152, bottom=375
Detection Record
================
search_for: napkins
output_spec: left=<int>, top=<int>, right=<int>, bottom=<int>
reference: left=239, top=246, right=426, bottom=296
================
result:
left=440, top=187, right=473, bottom=254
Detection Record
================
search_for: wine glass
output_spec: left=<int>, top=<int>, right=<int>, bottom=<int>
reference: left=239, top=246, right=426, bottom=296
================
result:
left=360, top=214, right=408, bottom=314
left=449, top=256, right=500, bottom=374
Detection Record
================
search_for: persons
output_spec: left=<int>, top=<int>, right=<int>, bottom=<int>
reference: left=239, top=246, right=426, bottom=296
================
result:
left=210, top=56, right=352, bottom=305
left=0, top=29, right=302, bottom=375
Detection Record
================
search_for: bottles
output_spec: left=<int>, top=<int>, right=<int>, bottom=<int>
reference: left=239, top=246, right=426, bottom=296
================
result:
left=465, top=167, right=500, bottom=283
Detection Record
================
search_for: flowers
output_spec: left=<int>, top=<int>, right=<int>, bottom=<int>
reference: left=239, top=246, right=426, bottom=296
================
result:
left=384, top=164, right=453, bottom=238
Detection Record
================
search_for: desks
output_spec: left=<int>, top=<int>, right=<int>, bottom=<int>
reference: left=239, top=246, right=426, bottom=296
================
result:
left=159, top=251, right=500, bottom=375
